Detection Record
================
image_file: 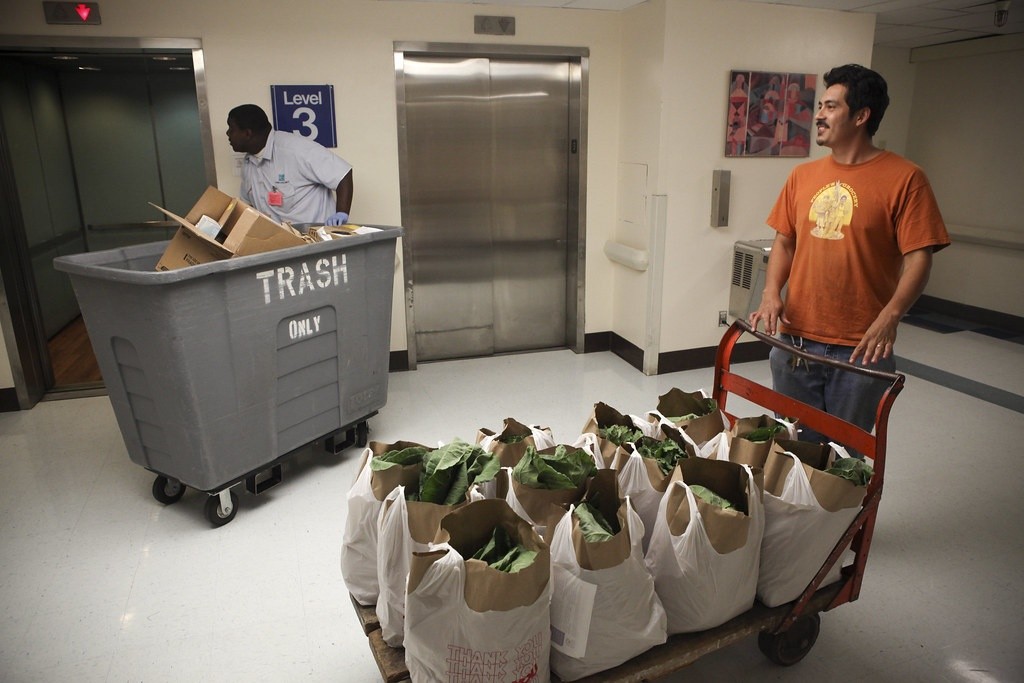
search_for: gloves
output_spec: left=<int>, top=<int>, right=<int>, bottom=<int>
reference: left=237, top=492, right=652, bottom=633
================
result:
left=325, top=211, right=348, bottom=227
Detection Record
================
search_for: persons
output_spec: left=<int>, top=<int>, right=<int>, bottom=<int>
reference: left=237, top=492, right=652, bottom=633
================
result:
left=225, top=104, right=353, bottom=226
left=748, top=64, right=953, bottom=461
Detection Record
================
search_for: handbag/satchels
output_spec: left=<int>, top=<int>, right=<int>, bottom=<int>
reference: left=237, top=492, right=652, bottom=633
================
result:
left=338, top=383, right=874, bottom=683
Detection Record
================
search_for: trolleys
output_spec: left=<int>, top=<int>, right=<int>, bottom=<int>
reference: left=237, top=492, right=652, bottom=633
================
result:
left=350, top=316, right=905, bottom=682
